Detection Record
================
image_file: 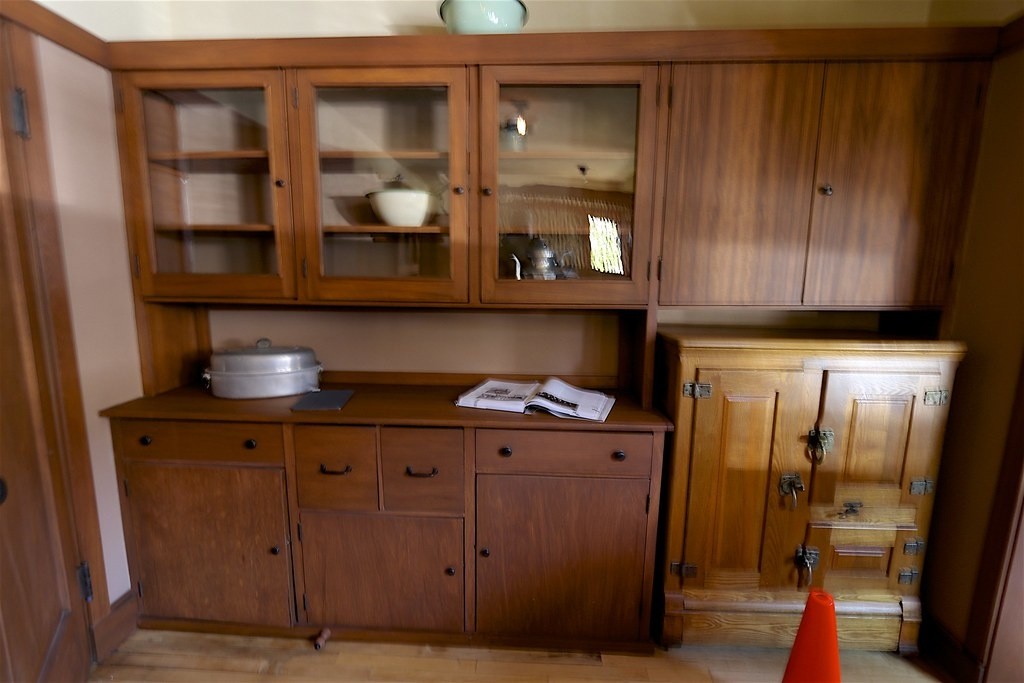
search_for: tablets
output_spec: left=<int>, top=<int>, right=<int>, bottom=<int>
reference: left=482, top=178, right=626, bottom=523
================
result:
left=291, top=390, right=355, bottom=409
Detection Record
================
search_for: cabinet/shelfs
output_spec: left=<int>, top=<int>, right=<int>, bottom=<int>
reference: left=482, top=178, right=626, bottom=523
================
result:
left=652, top=324, right=969, bottom=656
left=657, top=59, right=992, bottom=311
left=113, top=64, right=660, bottom=308
left=99, top=382, right=674, bottom=654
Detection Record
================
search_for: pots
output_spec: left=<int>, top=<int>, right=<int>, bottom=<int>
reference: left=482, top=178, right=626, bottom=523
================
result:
left=200, top=338, right=325, bottom=400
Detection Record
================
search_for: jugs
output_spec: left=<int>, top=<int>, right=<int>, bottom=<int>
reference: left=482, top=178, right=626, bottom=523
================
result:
left=510, top=238, right=576, bottom=281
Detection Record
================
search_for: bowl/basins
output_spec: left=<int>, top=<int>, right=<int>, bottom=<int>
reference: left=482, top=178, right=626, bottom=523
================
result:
left=439, top=0, right=529, bottom=35
left=365, top=188, right=439, bottom=228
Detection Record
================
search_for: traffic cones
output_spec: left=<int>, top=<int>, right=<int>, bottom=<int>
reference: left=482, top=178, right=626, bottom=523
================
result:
left=780, top=591, right=843, bottom=683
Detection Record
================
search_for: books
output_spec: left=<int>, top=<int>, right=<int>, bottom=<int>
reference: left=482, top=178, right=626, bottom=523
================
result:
left=456, top=375, right=615, bottom=423
